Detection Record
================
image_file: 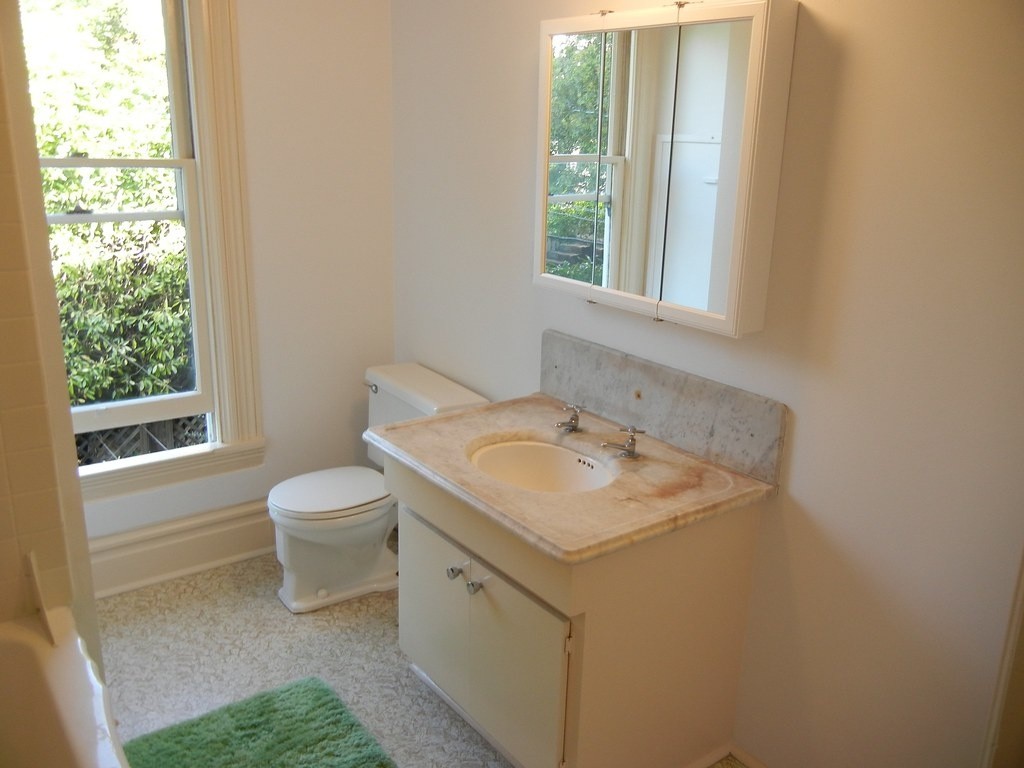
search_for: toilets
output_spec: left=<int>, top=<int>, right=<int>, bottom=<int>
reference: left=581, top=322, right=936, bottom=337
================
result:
left=267, top=361, right=493, bottom=614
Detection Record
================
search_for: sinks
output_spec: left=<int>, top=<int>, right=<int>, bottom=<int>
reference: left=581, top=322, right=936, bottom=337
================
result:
left=470, top=440, right=615, bottom=493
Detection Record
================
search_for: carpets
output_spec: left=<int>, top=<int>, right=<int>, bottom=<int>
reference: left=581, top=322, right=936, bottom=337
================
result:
left=122, top=675, right=398, bottom=768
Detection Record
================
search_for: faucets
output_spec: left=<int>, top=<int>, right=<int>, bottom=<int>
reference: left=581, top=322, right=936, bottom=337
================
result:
left=600, top=426, right=646, bottom=461
left=553, top=403, right=587, bottom=433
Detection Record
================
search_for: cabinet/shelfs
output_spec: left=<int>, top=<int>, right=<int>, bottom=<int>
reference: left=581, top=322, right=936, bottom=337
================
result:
left=383, top=471, right=765, bottom=768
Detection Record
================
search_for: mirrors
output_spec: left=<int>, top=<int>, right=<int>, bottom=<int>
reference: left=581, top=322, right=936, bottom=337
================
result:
left=528, top=2, right=799, bottom=339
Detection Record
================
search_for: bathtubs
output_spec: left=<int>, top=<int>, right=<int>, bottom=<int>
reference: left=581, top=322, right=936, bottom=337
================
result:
left=0, top=606, right=132, bottom=768
